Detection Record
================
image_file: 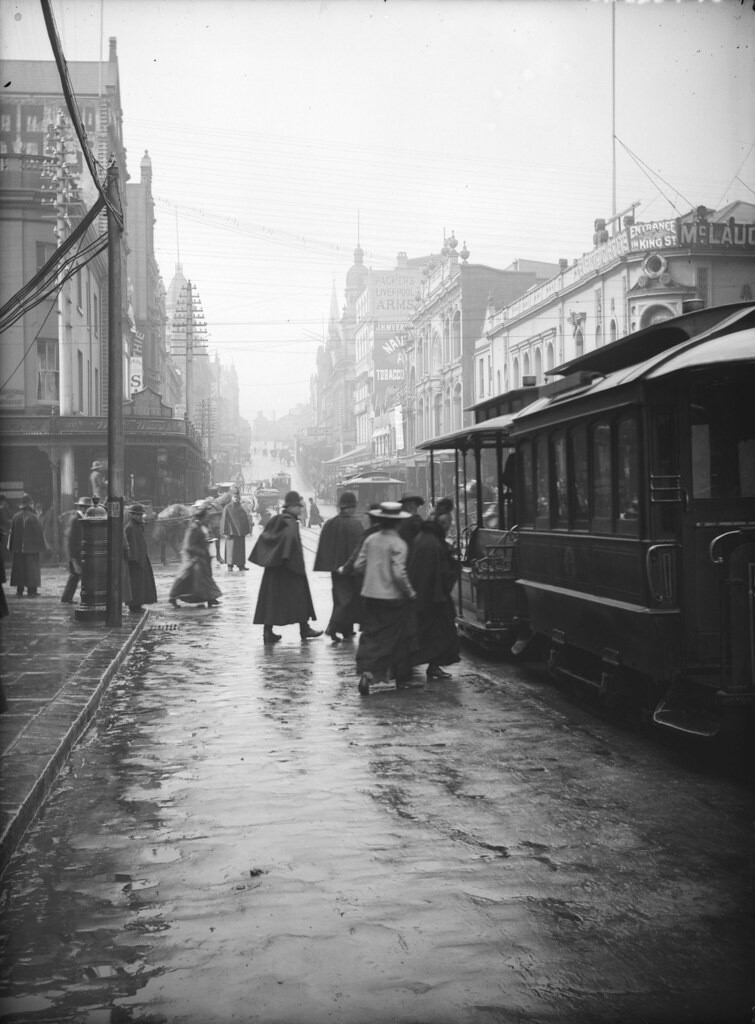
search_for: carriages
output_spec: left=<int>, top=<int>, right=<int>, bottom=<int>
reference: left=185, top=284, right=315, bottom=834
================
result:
left=58, top=474, right=253, bottom=568
left=255, top=479, right=282, bottom=516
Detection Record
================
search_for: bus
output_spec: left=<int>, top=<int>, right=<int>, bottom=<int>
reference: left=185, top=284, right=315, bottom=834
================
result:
left=334, top=470, right=408, bottom=530
left=272, top=468, right=291, bottom=498
left=415, top=300, right=755, bottom=754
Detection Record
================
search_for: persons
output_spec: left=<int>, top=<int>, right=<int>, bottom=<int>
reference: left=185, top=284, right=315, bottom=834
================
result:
left=0, top=494, right=12, bottom=532
left=219, top=494, right=251, bottom=571
left=337, top=495, right=425, bottom=595
left=247, top=491, right=324, bottom=643
left=354, top=502, right=425, bottom=695
left=90, top=461, right=108, bottom=498
left=101, top=496, right=132, bottom=603
left=168, top=509, right=223, bottom=608
left=124, top=505, right=157, bottom=613
left=401, top=497, right=463, bottom=678
left=299, top=496, right=308, bottom=527
left=307, top=497, right=325, bottom=528
left=252, top=501, right=271, bottom=528
left=33, top=500, right=46, bottom=516
left=61, top=497, right=92, bottom=604
left=312, top=492, right=364, bottom=642
left=6, top=493, right=47, bottom=595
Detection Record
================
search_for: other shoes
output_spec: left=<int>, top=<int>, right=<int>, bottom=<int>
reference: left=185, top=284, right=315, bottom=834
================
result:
left=358, top=676, right=369, bottom=696
left=264, top=633, right=282, bottom=644
left=16, top=593, right=23, bottom=597
left=427, top=667, right=452, bottom=678
left=300, top=629, right=323, bottom=640
left=325, top=627, right=341, bottom=640
left=228, top=567, right=233, bottom=571
left=61, top=599, right=78, bottom=604
left=208, top=600, right=222, bottom=607
left=397, top=678, right=423, bottom=689
left=28, top=593, right=41, bottom=597
left=239, top=567, right=249, bottom=571
left=344, top=632, right=356, bottom=636
left=169, top=599, right=181, bottom=608
left=131, top=608, right=147, bottom=614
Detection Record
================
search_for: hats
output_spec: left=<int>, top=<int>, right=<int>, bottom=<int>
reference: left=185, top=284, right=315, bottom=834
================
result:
left=368, top=503, right=382, bottom=523
left=365, top=502, right=413, bottom=519
left=281, top=491, right=303, bottom=507
left=90, top=461, right=102, bottom=469
left=398, top=496, right=424, bottom=505
left=129, top=505, right=146, bottom=514
left=335, top=493, right=357, bottom=509
left=74, top=497, right=93, bottom=506
left=18, top=494, right=35, bottom=508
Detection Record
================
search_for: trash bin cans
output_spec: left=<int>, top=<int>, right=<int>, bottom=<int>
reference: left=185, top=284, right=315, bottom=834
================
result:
left=74, top=494, right=112, bottom=620
